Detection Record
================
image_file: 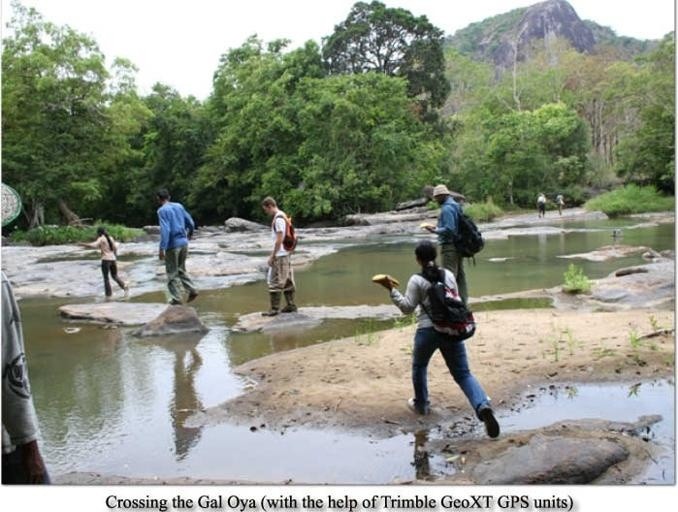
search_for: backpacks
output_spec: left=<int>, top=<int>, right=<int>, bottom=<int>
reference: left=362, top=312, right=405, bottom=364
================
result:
left=441, top=200, right=485, bottom=258
left=273, top=211, right=298, bottom=250
left=417, top=269, right=476, bottom=340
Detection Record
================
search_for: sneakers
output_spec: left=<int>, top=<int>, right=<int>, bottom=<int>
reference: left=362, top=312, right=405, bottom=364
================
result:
left=123, top=280, right=130, bottom=296
left=408, top=397, right=429, bottom=416
left=187, top=289, right=198, bottom=302
left=168, top=298, right=183, bottom=305
left=477, top=404, right=500, bottom=438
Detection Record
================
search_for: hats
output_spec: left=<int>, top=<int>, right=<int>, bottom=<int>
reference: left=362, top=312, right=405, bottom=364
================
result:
left=433, top=185, right=450, bottom=197
left=1, top=181, right=21, bottom=226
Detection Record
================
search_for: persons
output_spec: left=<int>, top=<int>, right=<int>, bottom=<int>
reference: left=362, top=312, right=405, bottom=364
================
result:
left=1, top=181, right=51, bottom=484
left=377, top=242, right=500, bottom=438
left=538, top=192, right=547, bottom=217
left=77, top=226, right=130, bottom=301
left=433, top=184, right=467, bottom=305
left=262, top=195, right=298, bottom=317
left=556, top=192, right=564, bottom=217
left=156, top=187, right=199, bottom=306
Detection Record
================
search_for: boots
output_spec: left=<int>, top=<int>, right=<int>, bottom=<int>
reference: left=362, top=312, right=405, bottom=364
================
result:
left=282, top=291, right=297, bottom=312
left=262, top=293, right=280, bottom=316
